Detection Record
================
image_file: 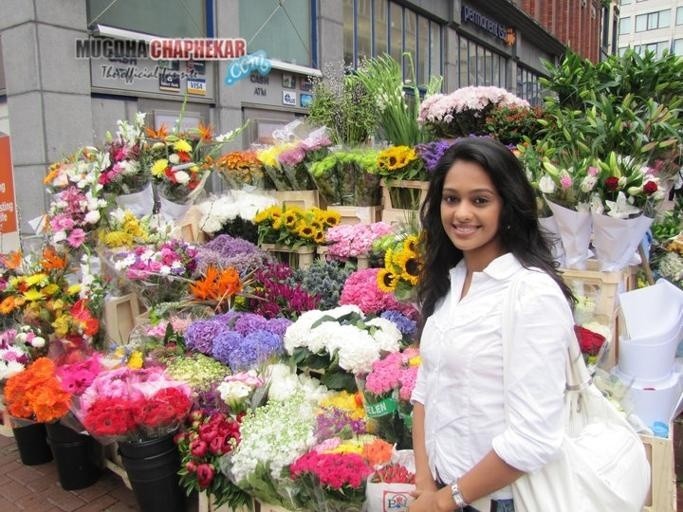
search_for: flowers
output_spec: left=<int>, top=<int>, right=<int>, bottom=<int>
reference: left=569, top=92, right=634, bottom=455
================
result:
left=0, top=48, right=683, bottom=511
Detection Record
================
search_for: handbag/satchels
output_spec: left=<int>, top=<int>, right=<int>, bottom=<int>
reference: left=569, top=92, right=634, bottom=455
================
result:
left=511, top=379, right=651, bottom=512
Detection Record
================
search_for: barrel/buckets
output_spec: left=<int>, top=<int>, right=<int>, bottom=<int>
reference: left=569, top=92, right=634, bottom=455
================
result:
left=9, top=413, right=54, bottom=466
left=116, top=431, right=192, bottom=511
left=45, top=423, right=101, bottom=490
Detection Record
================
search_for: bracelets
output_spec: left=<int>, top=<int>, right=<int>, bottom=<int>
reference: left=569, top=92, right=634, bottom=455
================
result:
left=449, top=480, right=469, bottom=510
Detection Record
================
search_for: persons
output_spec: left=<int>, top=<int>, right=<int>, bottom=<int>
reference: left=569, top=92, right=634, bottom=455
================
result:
left=404, top=136, right=575, bottom=512
